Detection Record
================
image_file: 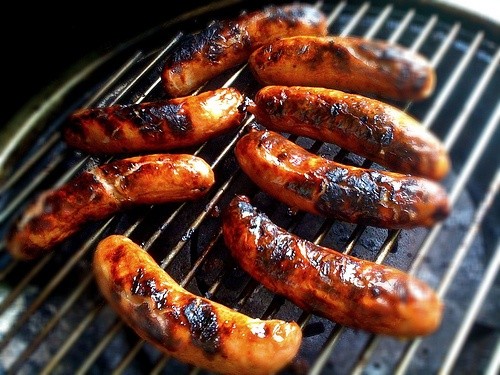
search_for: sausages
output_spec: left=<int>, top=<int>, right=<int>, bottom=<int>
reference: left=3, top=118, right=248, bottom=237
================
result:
left=158, top=5, right=327, bottom=98
left=247, top=36, right=437, bottom=101
left=246, top=84, right=449, bottom=180
left=57, top=88, right=247, bottom=153
left=5, top=153, right=215, bottom=259
left=91, top=235, right=303, bottom=375
left=223, top=195, right=442, bottom=336
left=235, top=123, right=449, bottom=226
